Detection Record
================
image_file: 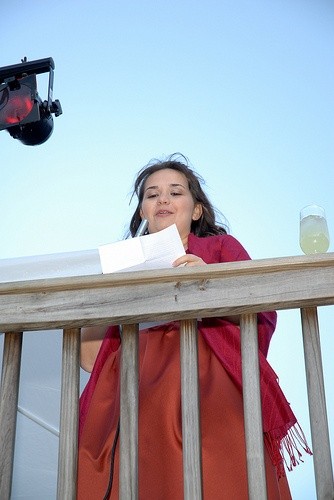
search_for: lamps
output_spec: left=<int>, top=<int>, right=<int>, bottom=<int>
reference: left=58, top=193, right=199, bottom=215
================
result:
left=0, top=56, right=64, bottom=147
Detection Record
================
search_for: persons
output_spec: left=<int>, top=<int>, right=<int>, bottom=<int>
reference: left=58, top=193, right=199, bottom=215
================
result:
left=79, top=154, right=313, bottom=499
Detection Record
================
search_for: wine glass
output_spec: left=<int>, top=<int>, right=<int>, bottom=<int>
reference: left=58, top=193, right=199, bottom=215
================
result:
left=298, top=204, right=330, bottom=253
